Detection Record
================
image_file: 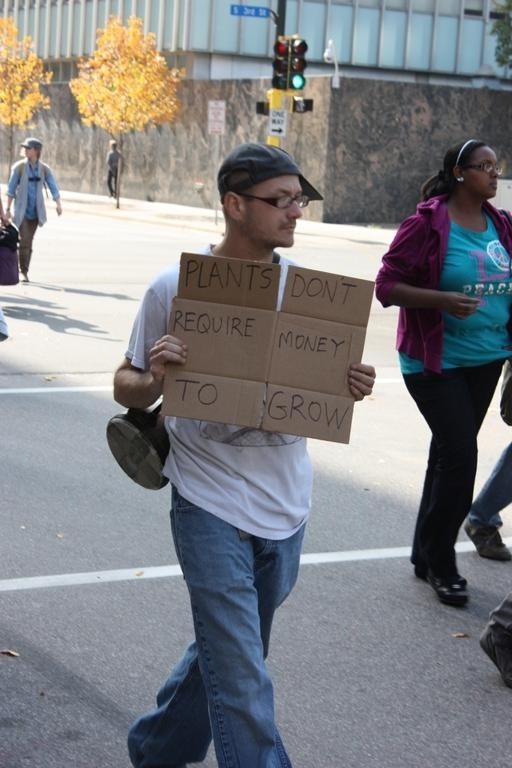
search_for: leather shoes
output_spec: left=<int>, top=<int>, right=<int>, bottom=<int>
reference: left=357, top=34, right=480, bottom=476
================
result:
left=414, top=563, right=467, bottom=589
left=428, top=568, right=469, bottom=605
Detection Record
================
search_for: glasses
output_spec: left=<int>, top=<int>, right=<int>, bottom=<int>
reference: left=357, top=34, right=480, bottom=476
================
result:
left=237, top=192, right=310, bottom=210
left=460, top=161, right=502, bottom=177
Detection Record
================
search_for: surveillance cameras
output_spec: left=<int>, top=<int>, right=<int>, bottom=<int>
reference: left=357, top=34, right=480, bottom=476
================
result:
left=324, top=49, right=334, bottom=64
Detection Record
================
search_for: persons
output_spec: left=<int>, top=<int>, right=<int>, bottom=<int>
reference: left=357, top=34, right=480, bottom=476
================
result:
left=0, top=195, right=14, bottom=227
left=6, top=137, right=62, bottom=284
left=114, top=142, right=375, bottom=766
left=376, top=139, right=512, bottom=605
left=104, top=140, right=123, bottom=198
left=463, top=358, right=512, bottom=562
left=478, top=590, right=512, bottom=689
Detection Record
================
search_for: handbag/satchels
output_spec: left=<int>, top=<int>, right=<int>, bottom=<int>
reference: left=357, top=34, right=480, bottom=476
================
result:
left=107, top=406, right=171, bottom=490
left=0, top=221, right=20, bottom=251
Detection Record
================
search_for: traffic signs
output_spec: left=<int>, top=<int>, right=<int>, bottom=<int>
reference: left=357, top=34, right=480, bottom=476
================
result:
left=269, top=107, right=287, bottom=137
left=231, top=4, right=270, bottom=17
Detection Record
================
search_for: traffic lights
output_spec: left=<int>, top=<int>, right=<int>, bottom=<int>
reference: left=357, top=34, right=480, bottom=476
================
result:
left=290, top=36, right=307, bottom=88
left=255, top=101, right=268, bottom=115
left=293, top=98, right=314, bottom=114
left=272, top=36, right=287, bottom=91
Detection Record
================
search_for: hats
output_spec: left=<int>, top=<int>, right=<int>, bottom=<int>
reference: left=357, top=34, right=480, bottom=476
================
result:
left=218, top=142, right=324, bottom=202
left=20, top=137, right=42, bottom=150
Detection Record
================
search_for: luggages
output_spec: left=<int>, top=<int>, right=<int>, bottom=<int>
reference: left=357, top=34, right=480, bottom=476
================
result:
left=0, top=218, right=22, bottom=285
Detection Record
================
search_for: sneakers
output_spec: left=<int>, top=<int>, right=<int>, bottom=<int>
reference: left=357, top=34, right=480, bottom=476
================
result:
left=480, top=620, right=512, bottom=687
left=464, top=521, right=512, bottom=560
left=23, top=274, right=29, bottom=282
